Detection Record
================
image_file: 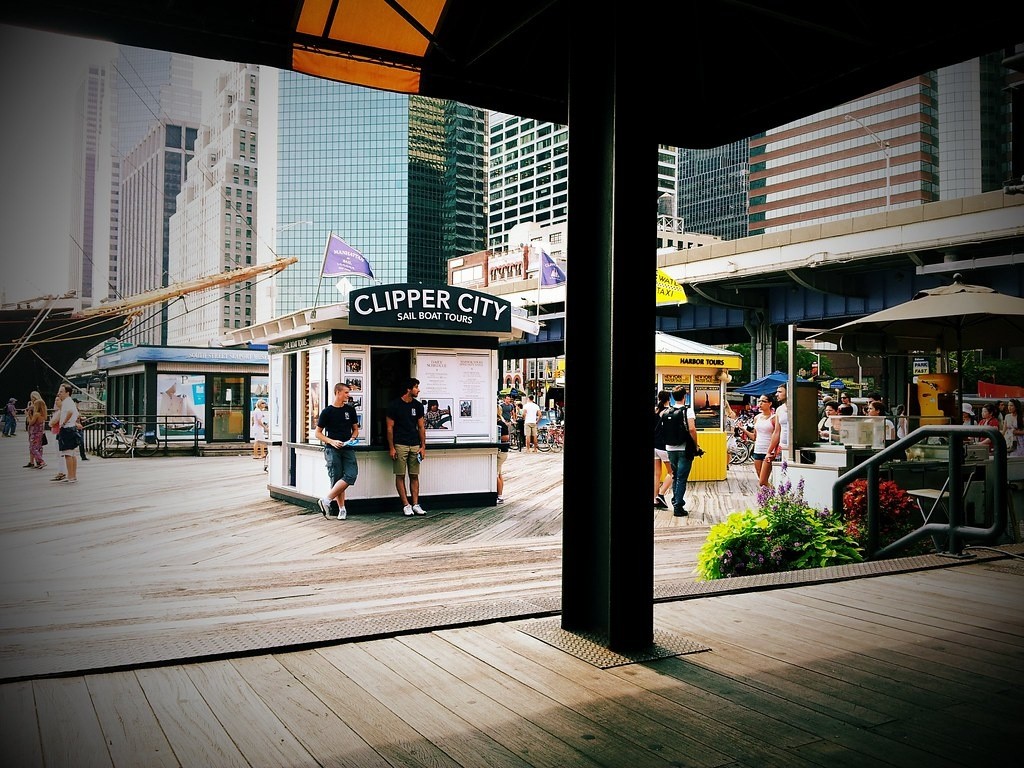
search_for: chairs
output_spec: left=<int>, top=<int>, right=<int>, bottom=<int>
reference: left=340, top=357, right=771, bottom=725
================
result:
left=906, top=464, right=977, bottom=553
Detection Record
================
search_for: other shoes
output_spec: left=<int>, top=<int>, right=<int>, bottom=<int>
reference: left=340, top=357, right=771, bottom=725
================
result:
left=4, top=434, right=11, bottom=437
left=337, top=510, right=347, bottom=520
left=34, top=465, right=41, bottom=469
left=82, top=458, right=89, bottom=460
left=62, top=479, right=75, bottom=483
left=253, top=455, right=266, bottom=459
left=11, top=433, right=16, bottom=436
left=39, top=463, right=47, bottom=469
left=75, top=478, right=77, bottom=481
left=23, top=463, right=34, bottom=468
left=497, top=497, right=503, bottom=504
left=318, top=499, right=331, bottom=520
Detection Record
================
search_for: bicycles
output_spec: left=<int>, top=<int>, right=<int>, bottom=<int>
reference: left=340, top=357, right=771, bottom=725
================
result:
left=726, top=417, right=754, bottom=465
left=97, top=413, right=159, bottom=458
left=509, top=419, right=565, bottom=453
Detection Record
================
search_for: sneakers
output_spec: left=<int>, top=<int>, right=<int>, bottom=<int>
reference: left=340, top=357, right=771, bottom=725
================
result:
left=656, top=494, right=668, bottom=508
left=674, top=510, right=688, bottom=516
left=654, top=503, right=659, bottom=507
left=403, top=504, right=414, bottom=516
left=413, top=505, right=427, bottom=515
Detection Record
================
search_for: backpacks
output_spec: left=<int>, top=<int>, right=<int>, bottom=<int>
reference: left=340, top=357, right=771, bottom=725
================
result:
left=2, top=404, right=13, bottom=415
left=656, top=407, right=688, bottom=446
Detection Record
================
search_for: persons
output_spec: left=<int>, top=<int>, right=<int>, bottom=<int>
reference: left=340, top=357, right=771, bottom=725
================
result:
left=654, top=385, right=697, bottom=516
left=23, top=391, right=48, bottom=469
left=2, top=398, right=17, bottom=437
left=962, top=399, right=1024, bottom=456
left=49, top=383, right=89, bottom=483
left=497, top=395, right=523, bottom=434
left=555, top=402, right=564, bottom=420
left=315, top=383, right=359, bottom=521
left=252, top=399, right=269, bottom=459
left=818, top=392, right=907, bottom=442
left=386, top=378, right=427, bottom=516
left=424, top=400, right=449, bottom=429
left=738, top=383, right=787, bottom=488
left=497, top=404, right=509, bottom=504
left=522, top=395, right=542, bottom=452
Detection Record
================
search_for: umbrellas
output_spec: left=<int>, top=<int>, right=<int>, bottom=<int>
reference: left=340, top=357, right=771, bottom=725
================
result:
left=809, top=374, right=863, bottom=401
left=806, top=273, right=1024, bottom=425
left=733, top=370, right=809, bottom=395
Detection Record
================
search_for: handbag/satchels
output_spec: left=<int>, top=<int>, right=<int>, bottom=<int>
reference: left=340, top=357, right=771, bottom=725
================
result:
left=42, top=434, right=48, bottom=445
left=684, top=410, right=699, bottom=461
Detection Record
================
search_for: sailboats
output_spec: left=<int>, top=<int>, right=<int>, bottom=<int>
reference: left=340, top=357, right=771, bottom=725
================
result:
left=0, top=58, right=298, bottom=413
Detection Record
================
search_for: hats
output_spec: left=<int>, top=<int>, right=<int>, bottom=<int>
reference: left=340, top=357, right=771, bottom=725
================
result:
left=10, top=398, right=18, bottom=402
left=962, top=403, right=975, bottom=416
left=72, top=398, right=82, bottom=403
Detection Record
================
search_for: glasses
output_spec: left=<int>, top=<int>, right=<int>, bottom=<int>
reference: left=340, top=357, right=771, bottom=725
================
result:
left=840, top=396, right=848, bottom=399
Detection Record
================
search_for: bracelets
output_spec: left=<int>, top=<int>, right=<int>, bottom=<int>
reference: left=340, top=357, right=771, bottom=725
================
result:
left=352, top=437, right=356, bottom=440
